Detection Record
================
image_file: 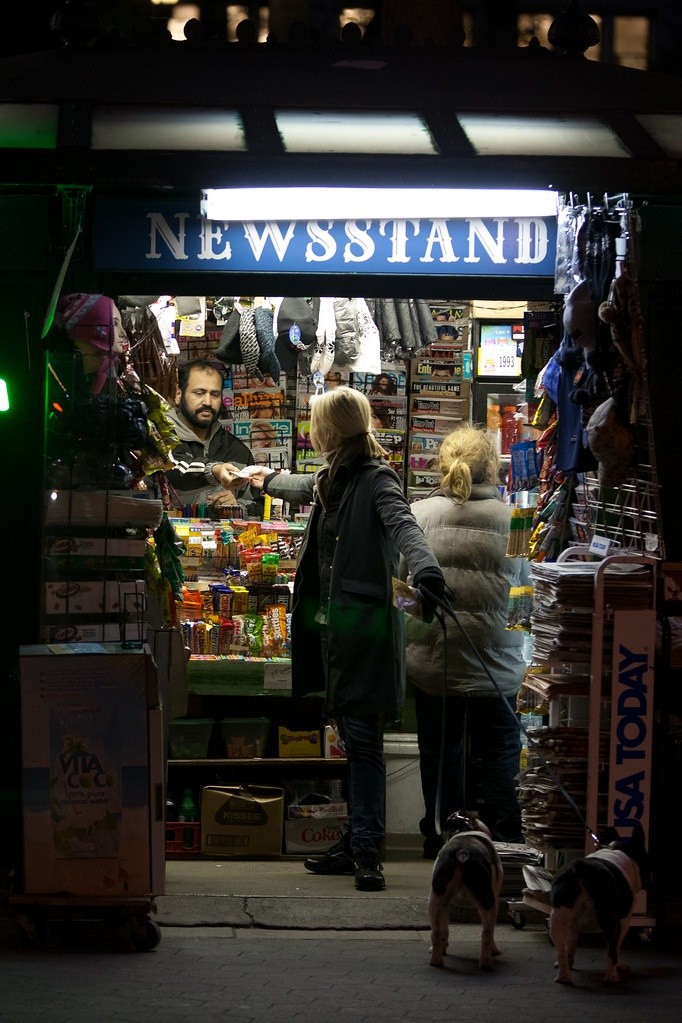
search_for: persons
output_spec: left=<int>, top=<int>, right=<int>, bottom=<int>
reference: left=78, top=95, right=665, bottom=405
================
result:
left=397, top=428, right=531, bottom=865
left=165, top=360, right=270, bottom=518
left=248, top=387, right=444, bottom=892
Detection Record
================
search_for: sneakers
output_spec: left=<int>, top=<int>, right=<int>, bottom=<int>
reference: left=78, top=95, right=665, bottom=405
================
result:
left=353, top=858, right=387, bottom=890
left=303, top=838, right=356, bottom=876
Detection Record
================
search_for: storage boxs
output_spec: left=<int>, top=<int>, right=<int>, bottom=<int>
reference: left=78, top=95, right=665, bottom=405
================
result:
left=164, top=820, right=201, bottom=859
left=285, top=801, right=347, bottom=856
left=201, top=785, right=286, bottom=861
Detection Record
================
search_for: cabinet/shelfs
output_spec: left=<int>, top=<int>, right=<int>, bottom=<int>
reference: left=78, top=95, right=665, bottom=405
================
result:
left=42, top=277, right=680, bottom=774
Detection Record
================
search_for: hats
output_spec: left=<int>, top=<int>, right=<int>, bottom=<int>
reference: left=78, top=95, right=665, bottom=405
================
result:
left=63, top=293, right=122, bottom=398
left=561, top=270, right=652, bottom=501
left=210, top=296, right=319, bottom=384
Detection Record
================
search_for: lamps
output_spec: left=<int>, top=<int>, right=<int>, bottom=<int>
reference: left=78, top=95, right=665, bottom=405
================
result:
left=200, top=183, right=559, bottom=225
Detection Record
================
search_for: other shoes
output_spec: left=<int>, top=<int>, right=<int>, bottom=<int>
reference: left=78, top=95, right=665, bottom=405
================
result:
left=422, top=836, right=446, bottom=860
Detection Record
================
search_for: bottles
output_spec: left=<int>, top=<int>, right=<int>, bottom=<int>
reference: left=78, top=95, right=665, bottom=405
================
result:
left=179, top=786, right=196, bottom=849
left=164, top=789, right=176, bottom=838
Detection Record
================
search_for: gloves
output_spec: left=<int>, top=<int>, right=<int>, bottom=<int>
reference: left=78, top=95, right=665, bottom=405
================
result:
left=412, top=566, right=446, bottom=625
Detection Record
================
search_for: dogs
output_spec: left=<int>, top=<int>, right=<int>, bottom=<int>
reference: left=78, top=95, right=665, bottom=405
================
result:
left=427, top=799, right=504, bottom=972
left=548, top=818, right=655, bottom=985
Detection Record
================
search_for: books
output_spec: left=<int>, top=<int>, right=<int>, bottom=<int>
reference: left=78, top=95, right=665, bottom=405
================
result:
left=159, top=308, right=467, bottom=518
left=517, top=559, right=653, bottom=911
